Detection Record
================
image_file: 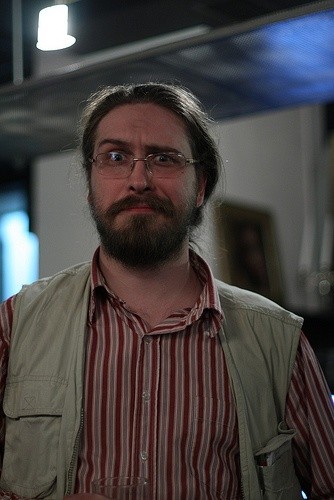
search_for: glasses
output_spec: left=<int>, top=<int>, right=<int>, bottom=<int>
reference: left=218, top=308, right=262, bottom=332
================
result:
left=88, top=152, right=201, bottom=180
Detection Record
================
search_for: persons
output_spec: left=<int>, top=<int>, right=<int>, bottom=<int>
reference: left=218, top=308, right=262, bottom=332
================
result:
left=0, top=81, right=334, bottom=500
